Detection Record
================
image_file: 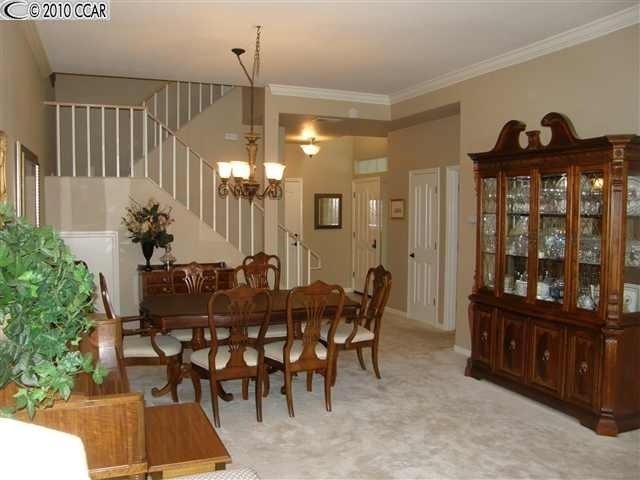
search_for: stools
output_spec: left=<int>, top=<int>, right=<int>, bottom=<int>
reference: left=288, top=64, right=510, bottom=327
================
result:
left=137, top=402, right=233, bottom=480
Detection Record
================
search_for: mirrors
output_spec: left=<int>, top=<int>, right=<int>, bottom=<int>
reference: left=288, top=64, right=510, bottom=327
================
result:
left=314, top=194, right=343, bottom=229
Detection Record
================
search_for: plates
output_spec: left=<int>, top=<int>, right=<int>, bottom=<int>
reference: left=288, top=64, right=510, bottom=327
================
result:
left=625, top=176, right=640, bottom=215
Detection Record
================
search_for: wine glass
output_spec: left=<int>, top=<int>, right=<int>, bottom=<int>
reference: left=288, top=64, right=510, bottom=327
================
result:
left=485, top=219, right=639, bottom=264
left=481, top=174, right=602, bottom=214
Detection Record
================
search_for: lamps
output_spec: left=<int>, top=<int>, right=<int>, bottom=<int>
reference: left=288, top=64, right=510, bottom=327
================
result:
left=298, top=136, right=321, bottom=158
left=215, top=25, right=286, bottom=206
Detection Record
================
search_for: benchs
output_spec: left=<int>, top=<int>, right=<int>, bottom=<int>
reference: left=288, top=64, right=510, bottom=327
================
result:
left=0, top=313, right=148, bottom=480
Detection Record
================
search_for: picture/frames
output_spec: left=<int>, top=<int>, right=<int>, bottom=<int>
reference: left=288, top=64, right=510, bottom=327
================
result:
left=391, top=199, right=405, bottom=219
left=0, top=131, right=40, bottom=231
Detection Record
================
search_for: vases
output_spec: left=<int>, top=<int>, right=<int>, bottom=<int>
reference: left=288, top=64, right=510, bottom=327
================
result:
left=142, top=244, right=154, bottom=272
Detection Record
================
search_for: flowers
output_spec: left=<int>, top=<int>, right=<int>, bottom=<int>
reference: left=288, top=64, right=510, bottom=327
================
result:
left=123, top=193, right=175, bottom=249
